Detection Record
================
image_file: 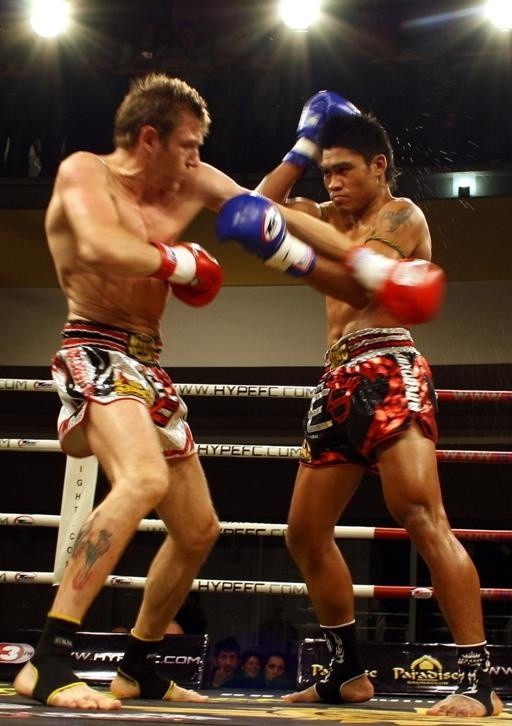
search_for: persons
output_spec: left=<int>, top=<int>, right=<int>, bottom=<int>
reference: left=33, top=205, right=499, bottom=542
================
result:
left=262, top=654, right=286, bottom=683
left=163, top=619, right=184, bottom=633
left=205, top=640, right=241, bottom=689
left=7, top=71, right=446, bottom=712
left=249, top=88, right=503, bottom=717
left=241, top=652, right=262, bottom=679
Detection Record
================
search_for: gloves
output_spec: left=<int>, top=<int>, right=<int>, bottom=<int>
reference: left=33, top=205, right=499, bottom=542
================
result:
left=214, top=192, right=315, bottom=280
left=147, top=239, right=223, bottom=307
left=281, top=90, right=363, bottom=172
left=340, top=243, right=446, bottom=327
